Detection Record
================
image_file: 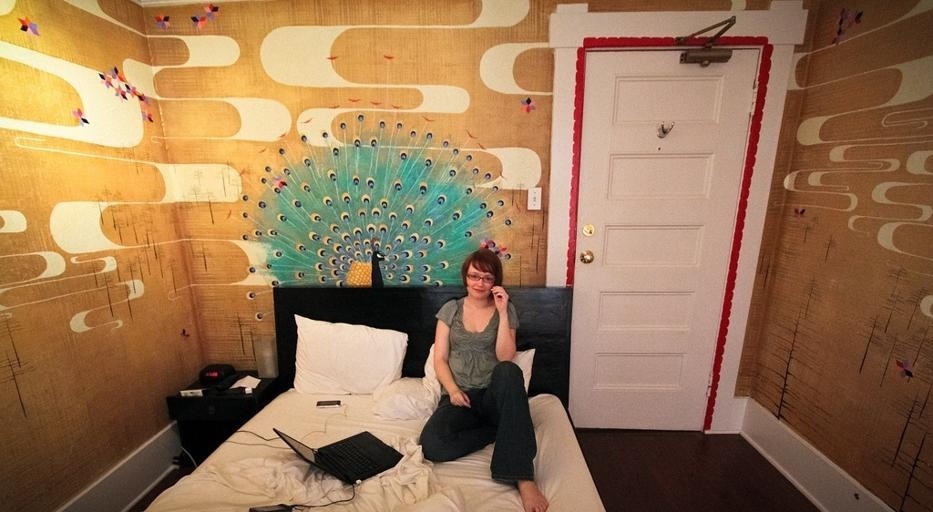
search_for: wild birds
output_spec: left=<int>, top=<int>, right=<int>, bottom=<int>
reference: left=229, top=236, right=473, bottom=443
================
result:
left=237, top=114, right=515, bottom=322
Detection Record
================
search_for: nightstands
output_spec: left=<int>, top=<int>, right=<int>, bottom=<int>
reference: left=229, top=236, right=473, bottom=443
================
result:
left=164, top=377, right=275, bottom=470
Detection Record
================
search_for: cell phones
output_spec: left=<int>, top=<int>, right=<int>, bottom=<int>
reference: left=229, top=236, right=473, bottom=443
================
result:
left=316, top=400, right=341, bottom=407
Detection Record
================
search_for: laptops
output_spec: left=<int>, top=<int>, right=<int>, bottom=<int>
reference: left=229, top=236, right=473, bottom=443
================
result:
left=272, top=427, right=404, bottom=484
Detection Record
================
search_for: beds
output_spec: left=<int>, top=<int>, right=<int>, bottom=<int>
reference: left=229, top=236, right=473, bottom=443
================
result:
left=144, top=286, right=607, bottom=510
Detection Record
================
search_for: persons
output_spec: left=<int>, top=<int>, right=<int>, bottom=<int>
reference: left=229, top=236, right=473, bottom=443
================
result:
left=418, top=249, right=549, bottom=512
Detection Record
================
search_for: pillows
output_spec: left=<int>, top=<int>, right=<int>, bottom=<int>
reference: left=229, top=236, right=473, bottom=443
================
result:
left=425, top=344, right=536, bottom=397
left=293, top=314, right=410, bottom=395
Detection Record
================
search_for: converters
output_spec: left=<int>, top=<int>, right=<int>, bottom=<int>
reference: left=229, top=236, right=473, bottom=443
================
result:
left=249, top=504, right=292, bottom=512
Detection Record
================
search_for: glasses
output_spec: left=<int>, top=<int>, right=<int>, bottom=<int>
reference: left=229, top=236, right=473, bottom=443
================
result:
left=467, top=274, right=494, bottom=283
